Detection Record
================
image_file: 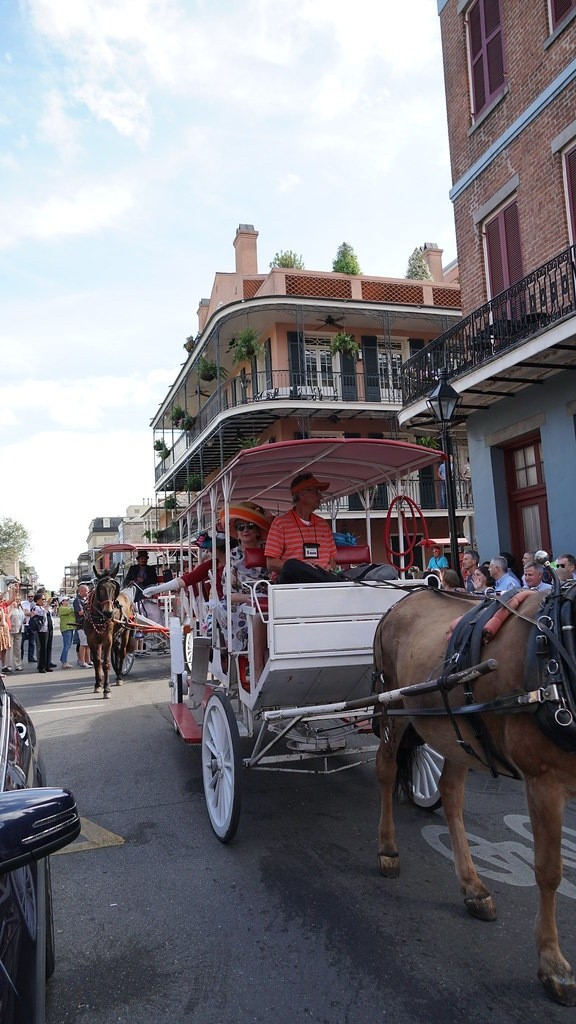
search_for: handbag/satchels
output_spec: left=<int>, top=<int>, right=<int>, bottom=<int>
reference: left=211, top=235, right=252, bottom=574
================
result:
left=28, top=616, right=45, bottom=632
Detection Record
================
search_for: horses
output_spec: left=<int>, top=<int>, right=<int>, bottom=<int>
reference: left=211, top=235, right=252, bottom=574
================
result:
left=374, top=586, right=576, bottom=1006
left=83, top=562, right=134, bottom=699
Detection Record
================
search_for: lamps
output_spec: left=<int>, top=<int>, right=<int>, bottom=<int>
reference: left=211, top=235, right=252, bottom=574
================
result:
left=240, top=375, right=251, bottom=388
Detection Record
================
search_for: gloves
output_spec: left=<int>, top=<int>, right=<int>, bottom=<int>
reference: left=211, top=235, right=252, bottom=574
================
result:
left=143, top=579, right=179, bottom=598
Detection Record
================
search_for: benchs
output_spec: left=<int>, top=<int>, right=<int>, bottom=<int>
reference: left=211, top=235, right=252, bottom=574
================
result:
left=182, top=545, right=398, bottom=616
left=142, top=576, right=176, bottom=607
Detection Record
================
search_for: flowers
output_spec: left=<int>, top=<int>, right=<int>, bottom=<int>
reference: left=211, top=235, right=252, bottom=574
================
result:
left=256, top=506, right=273, bottom=521
left=176, top=416, right=194, bottom=431
left=196, top=529, right=225, bottom=547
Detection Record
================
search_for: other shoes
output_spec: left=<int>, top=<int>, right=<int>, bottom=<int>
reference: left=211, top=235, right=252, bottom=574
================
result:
left=62, top=664, right=74, bottom=669
left=38, top=669, right=46, bottom=673
left=2, top=668, right=12, bottom=673
left=45, top=667, right=53, bottom=672
left=88, top=661, right=94, bottom=665
left=15, top=666, right=23, bottom=671
left=50, top=663, right=57, bottom=667
left=28, top=658, right=37, bottom=662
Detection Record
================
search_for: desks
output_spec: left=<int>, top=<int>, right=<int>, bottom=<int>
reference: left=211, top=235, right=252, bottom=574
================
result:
left=476, top=318, right=519, bottom=352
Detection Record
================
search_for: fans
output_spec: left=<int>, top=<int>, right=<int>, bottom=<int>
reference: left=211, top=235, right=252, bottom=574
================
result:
left=190, top=389, right=212, bottom=398
left=314, top=314, right=346, bottom=330
left=224, top=338, right=239, bottom=354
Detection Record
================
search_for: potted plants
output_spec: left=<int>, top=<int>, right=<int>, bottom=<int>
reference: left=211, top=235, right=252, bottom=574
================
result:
left=184, top=475, right=207, bottom=492
left=417, top=434, right=441, bottom=450
left=231, top=325, right=268, bottom=362
left=195, top=356, right=230, bottom=382
left=329, top=329, right=361, bottom=360
left=236, top=435, right=262, bottom=450
left=141, top=528, right=166, bottom=539
left=154, top=438, right=170, bottom=460
left=164, top=494, right=181, bottom=510
left=184, top=336, right=195, bottom=353
left=169, top=404, right=188, bottom=427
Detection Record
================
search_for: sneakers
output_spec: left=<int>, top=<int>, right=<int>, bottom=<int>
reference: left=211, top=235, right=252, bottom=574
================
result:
left=80, top=663, right=92, bottom=669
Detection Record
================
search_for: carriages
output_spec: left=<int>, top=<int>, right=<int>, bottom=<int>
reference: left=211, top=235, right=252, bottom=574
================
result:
left=146, top=294, right=575, bottom=1024
left=77, top=541, right=197, bottom=702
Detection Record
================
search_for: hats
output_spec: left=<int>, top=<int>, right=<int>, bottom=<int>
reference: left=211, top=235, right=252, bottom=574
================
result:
left=58, top=596, right=70, bottom=603
left=136, top=551, right=149, bottom=560
left=220, top=502, right=272, bottom=541
left=187, top=519, right=234, bottom=549
left=291, top=478, right=330, bottom=492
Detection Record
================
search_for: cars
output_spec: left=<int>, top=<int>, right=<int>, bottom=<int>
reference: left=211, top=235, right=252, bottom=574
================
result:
left=0, top=671, right=84, bottom=1024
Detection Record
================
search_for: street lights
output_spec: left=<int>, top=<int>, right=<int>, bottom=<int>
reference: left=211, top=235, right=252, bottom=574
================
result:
left=424, top=369, right=465, bottom=585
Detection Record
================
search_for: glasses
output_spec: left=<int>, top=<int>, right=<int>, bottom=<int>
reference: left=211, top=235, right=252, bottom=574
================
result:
left=308, top=488, right=319, bottom=494
left=28, top=596, right=33, bottom=598
left=41, top=599, right=46, bottom=601
left=556, top=564, right=565, bottom=568
left=433, top=551, right=438, bottom=553
left=235, top=522, right=254, bottom=531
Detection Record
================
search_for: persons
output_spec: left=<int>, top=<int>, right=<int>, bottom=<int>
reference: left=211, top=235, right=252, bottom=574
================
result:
left=437, top=455, right=474, bottom=509
left=143, top=473, right=345, bottom=687
left=405, top=546, right=576, bottom=595
left=0, top=576, right=93, bottom=674
left=122, top=551, right=158, bottom=618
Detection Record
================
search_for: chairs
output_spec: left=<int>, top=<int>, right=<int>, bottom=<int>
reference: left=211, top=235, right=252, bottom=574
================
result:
left=252, top=385, right=341, bottom=401
